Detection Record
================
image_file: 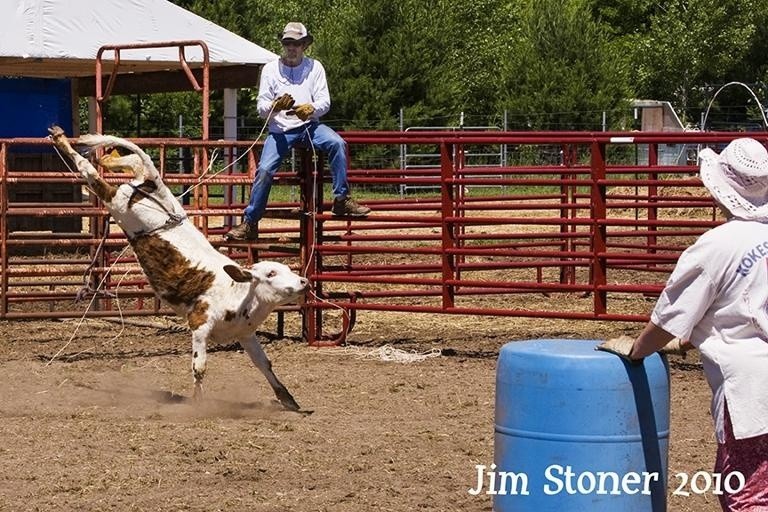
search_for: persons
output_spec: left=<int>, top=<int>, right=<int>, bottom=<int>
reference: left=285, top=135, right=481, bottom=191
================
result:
left=227, top=20, right=371, bottom=240
left=596, top=138, right=767, bottom=511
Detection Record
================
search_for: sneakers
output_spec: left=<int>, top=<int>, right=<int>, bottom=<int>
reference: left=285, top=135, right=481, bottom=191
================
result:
left=225, top=220, right=259, bottom=242
left=331, top=194, right=371, bottom=217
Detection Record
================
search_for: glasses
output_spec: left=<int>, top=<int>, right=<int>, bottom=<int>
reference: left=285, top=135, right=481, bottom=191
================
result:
left=282, top=40, right=305, bottom=48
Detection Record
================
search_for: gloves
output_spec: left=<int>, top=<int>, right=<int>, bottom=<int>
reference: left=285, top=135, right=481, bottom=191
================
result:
left=657, top=331, right=688, bottom=360
left=286, top=102, right=315, bottom=122
left=272, top=92, right=296, bottom=112
left=594, top=335, right=645, bottom=365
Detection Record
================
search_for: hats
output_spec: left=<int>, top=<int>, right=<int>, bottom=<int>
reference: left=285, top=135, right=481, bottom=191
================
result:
left=277, top=21, right=314, bottom=45
left=697, top=136, right=768, bottom=223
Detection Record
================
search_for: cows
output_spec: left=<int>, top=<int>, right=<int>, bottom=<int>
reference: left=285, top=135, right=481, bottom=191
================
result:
left=45, top=122, right=311, bottom=413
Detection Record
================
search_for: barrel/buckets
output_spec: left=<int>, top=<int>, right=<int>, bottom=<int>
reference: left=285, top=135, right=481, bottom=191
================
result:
left=492, top=338, right=671, bottom=512
